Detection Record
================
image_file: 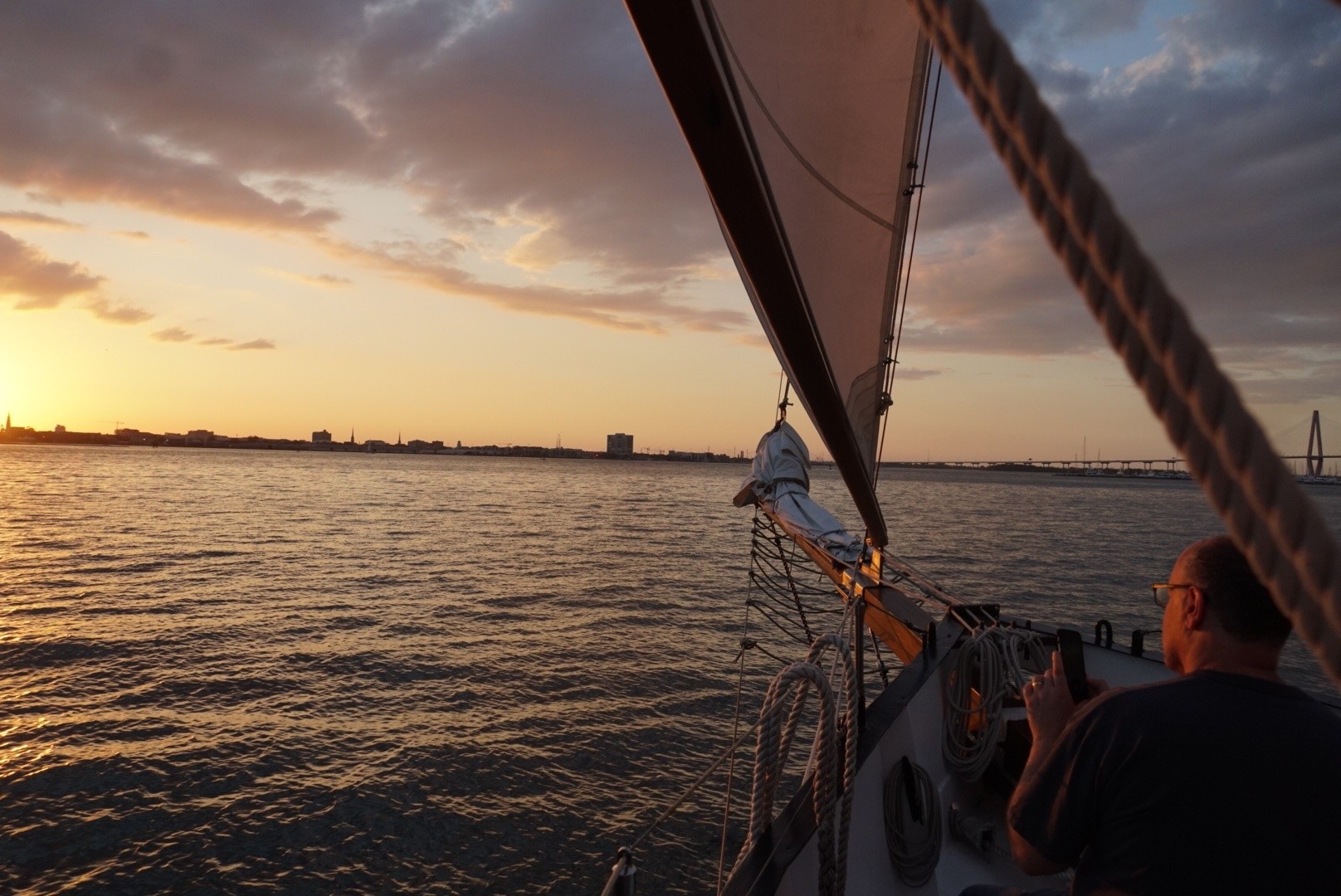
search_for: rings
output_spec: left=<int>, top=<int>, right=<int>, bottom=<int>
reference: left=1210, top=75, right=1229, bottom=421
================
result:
left=1031, top=682, right=1042, bottom=686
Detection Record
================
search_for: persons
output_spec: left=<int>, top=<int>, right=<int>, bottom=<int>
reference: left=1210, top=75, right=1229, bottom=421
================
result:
left=1007, top=534, right=1341, bottom=896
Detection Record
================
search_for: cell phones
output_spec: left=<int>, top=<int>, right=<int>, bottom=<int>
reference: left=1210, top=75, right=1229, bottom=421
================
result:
left=1056, top=628, right=1090, bottom=703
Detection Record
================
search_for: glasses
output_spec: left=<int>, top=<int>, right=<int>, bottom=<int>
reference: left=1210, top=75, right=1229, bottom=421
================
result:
left=1151, top=584, right=1220, bottom=609
